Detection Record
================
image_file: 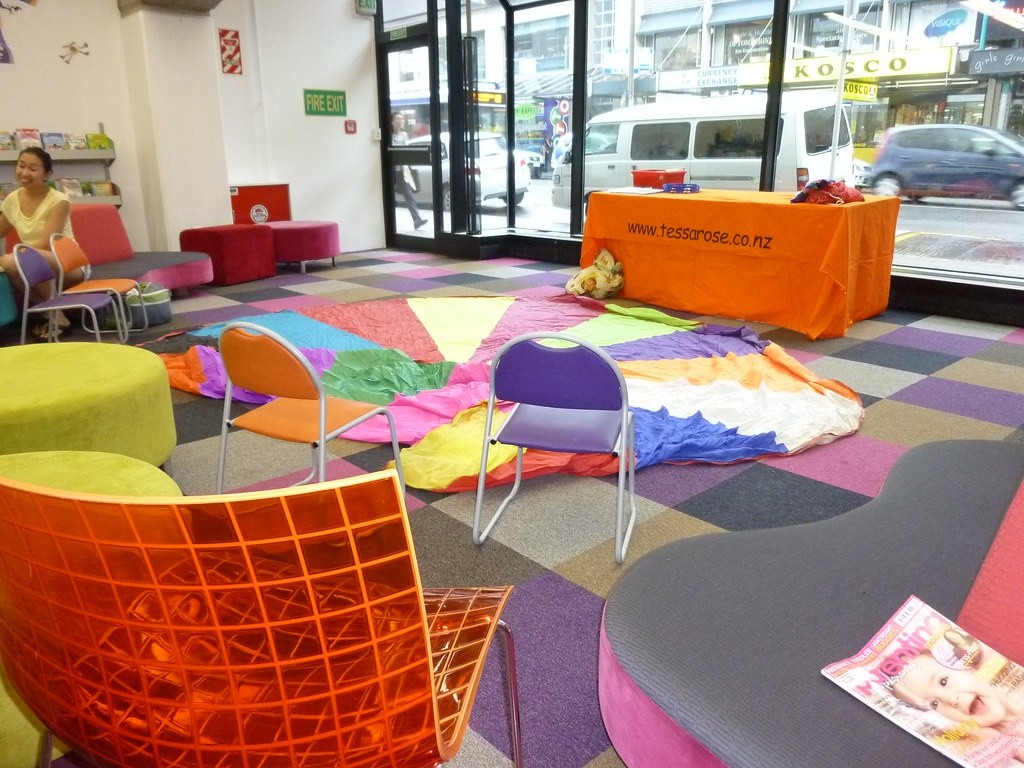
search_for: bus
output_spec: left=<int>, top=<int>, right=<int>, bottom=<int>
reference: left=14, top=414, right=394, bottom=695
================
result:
left=390, top=81, right=508, bottom=140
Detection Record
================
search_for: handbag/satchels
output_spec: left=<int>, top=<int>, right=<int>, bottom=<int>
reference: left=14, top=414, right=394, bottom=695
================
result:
left=96, top=282, right=172, bottom=330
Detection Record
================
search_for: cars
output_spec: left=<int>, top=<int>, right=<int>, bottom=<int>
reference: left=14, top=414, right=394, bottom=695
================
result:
left=396, top=132, right=530, bottom=212
left=521, top=151, right=544, bottom=179
left=869, top=124, right=1024, bottom=211
left=854, top=158, right=873, bottom=190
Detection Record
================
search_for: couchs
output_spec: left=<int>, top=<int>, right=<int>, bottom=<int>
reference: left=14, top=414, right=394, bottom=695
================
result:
left=597, top=439, right=1024, bottom=768
left=0, top=451, right=185, bottom=768
left=4, top=203, right=214, bottom=300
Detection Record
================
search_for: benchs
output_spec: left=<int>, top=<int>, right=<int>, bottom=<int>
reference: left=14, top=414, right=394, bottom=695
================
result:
left=0, top=469, right=520, bottom=768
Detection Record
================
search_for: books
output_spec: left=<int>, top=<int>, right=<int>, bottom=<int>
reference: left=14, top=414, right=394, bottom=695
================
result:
left=821, top=594, right=1024, bottom=768
left=0, top=128, right=115, bottom=201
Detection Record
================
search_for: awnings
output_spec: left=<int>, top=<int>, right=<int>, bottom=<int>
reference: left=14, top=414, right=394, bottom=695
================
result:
left=499, top=67, right=604, bottom=98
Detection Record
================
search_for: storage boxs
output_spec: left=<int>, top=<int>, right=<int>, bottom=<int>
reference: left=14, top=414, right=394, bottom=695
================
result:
left=632, top=169, right=687, bottom=188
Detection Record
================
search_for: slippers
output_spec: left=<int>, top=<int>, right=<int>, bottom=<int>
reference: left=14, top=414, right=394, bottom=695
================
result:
left=31, top=322, right=73, bottom=343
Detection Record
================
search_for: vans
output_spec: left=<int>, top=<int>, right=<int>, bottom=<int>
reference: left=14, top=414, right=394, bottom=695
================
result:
left=552, top=92, right=855, bottom=217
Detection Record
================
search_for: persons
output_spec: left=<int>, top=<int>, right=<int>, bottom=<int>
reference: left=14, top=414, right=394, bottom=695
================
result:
left=885, top=644, right=1023, bottom=764
left=391, top=112, right=428, bottom=229
left=0, top=147, right=91, bottom=343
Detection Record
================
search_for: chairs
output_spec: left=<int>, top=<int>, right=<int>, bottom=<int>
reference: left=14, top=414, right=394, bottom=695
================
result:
left=214, top=321, right=406, bottom=507
left=14, top=244, right=124, bottom=344
left=49, top=233, right=149, bottom=344
left=473, top=332, right=636, bottom=566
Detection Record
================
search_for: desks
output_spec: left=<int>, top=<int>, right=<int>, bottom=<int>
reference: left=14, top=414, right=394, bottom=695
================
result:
left=580, top=187, right=901, bottom=339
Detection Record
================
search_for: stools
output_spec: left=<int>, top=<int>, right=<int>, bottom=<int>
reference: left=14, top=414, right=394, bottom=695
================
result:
left=179, top=224, right=275, bottom=288
left=0, top=343, right=178, bottom=477
left=253, top=221, right=340, bottom=275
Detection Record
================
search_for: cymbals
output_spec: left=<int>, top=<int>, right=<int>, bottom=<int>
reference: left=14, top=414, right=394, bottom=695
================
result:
left=663, top=183, right=700, bottom=194
